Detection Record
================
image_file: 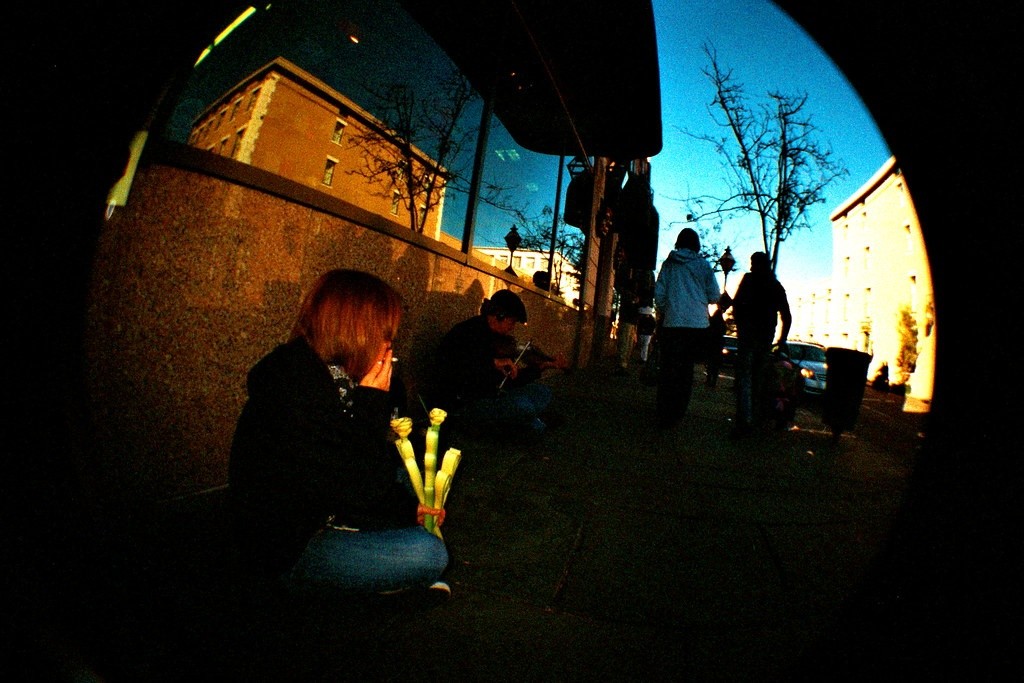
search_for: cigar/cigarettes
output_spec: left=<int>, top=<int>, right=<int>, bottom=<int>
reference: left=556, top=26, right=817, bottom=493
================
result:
left=383, top=357, right=399, bottom=362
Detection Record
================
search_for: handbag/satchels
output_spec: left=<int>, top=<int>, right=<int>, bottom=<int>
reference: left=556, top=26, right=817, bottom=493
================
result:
left=753, top=358, right=800, bottom=403
left=639, top=366, right=656, bottom=384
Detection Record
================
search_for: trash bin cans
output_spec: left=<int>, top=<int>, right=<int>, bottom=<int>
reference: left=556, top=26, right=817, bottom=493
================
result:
left=821, top=349, right=874, bottom=434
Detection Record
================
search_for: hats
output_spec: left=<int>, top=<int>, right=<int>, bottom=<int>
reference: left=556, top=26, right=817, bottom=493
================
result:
left=487, top=289, right=528, bottom=322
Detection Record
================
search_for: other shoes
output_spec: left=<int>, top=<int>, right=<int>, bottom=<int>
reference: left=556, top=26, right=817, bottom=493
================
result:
left=376, top=581, right=411, bottom=594
left=428, top=575, right=452, bottom=601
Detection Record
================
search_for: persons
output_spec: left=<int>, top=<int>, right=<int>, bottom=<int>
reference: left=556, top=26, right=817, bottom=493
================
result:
left=225, top=271, right=451, bottom=607
left=437, top=286, right=567, bottom=438
left=733, top=252, right=792, bottom=438
left=619, top=228, right=721, bottom=428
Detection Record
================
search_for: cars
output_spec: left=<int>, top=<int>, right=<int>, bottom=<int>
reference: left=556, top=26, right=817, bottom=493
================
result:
left=719, top=334, right=739, bottom=368
left=771, top=341, right=828, bottom=399
